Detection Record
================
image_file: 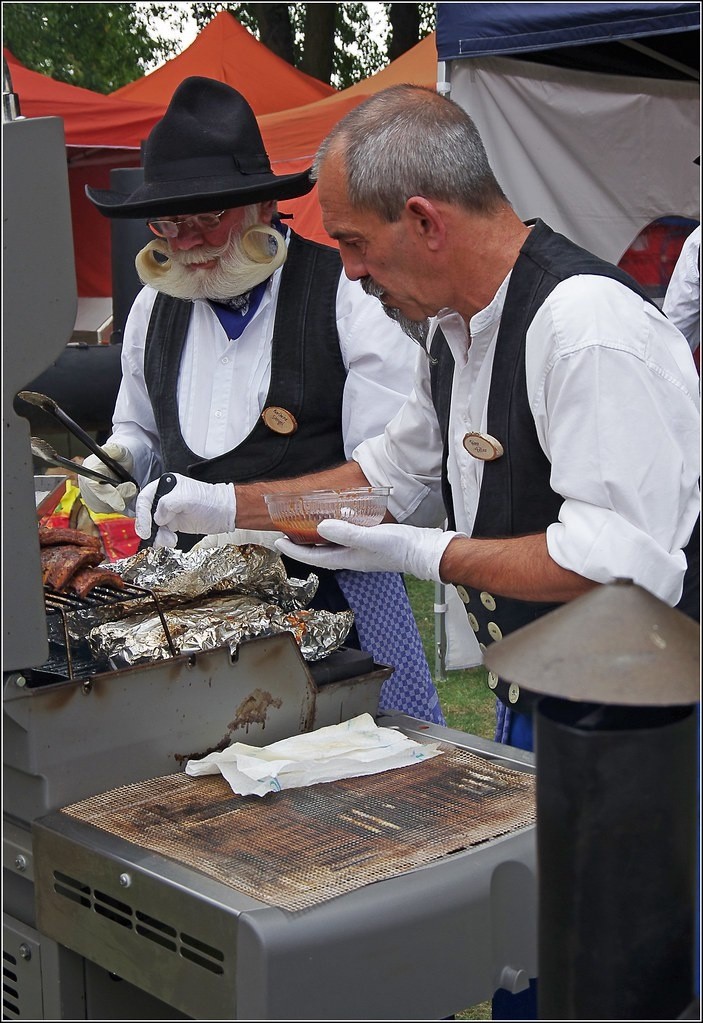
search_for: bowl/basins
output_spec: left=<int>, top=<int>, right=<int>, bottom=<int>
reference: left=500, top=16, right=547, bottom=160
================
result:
left=263, top=485, right=393, bottom=546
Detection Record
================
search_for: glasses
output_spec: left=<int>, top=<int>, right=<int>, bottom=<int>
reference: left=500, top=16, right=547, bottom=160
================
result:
left=145, top=211, right=228, bottom=239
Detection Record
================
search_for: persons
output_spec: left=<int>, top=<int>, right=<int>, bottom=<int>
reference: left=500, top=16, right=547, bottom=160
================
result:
left=136, top=83, right=703, bottom=754
left=79, top=75, right=445, bottom=730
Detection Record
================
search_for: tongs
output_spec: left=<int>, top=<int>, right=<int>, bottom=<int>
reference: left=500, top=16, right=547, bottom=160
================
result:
left=19, top=390, right=139, bottom=497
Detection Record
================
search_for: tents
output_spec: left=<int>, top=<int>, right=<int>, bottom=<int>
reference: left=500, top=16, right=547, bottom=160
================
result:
left=4, top=2, right=701, bottom=332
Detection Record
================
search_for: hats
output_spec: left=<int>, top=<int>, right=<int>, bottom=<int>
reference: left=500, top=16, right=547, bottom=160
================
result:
left=85, top=76, right=316, bottom=219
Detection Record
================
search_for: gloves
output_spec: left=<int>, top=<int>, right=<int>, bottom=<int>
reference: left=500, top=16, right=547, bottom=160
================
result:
left=276, top=515, right=472, bottom=587
left=78, top=442, right=138, bottom=519
left=134, top=472, right=239, bottom=540
